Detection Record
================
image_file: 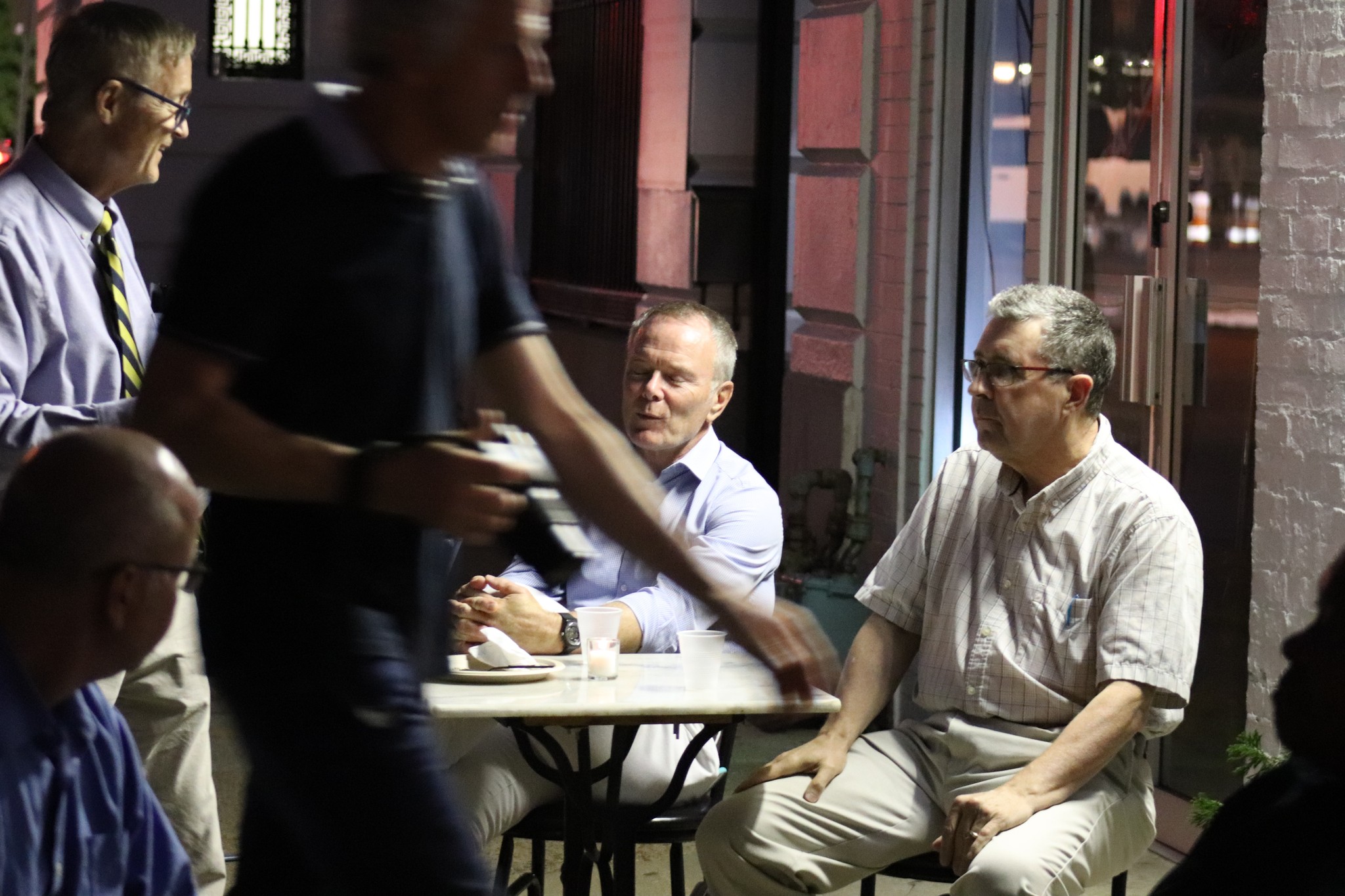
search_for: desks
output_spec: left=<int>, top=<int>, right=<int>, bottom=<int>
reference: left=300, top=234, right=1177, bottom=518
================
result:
left=420, top=653, right=842, bottom=896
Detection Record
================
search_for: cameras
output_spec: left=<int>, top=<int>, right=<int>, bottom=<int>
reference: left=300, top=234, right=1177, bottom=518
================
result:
left=455, top=419, right=596, bottom=587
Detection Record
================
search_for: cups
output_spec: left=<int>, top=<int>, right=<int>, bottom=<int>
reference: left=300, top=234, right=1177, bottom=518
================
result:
left=574, top=606, right=623, bottom=665
left=587, top=637, right=621, bottom=681
left=675, top=630, right=728, bottom=686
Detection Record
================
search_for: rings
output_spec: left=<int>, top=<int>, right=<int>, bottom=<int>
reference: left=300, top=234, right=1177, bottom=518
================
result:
left=969, top=829, right=979, bottom=838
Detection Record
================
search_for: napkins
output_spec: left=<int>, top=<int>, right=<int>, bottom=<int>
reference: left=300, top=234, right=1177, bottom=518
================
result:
left=468, top=626, right=540, bottom=673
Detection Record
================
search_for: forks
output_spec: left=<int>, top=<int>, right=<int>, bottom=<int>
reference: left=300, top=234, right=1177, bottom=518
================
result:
left=459, top=665, right=557, bottom=672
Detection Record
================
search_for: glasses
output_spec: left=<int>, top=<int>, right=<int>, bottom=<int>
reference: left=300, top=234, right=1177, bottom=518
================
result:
left=957, top=357, right=1075, bottom=389
left=92, top=76, right=195, bottom=126
left=99, top=561, right=208, bottom=595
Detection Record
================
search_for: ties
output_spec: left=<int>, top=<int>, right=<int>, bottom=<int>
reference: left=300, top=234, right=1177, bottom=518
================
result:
left=96, top=207, right=207, bottom=556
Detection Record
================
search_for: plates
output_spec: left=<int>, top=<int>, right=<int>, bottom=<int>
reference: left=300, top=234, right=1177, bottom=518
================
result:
left=447, top=654, right=566, bottom=684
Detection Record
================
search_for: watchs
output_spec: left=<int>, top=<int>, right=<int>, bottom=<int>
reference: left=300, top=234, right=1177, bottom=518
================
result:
left=560, top=612, right=582, bottom=654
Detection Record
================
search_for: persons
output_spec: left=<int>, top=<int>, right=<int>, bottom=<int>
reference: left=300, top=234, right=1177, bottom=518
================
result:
left=693, top=286, right=1205, bottom=896
left=1147, top=548, right=1345, bottom=896
left=413, top=305, right=782, bottom=846
left=0, top=423, right=199, bottom=896
left=129, top=0, right=833, bottom=896
left=0, top=3, right=227, bottom=896
